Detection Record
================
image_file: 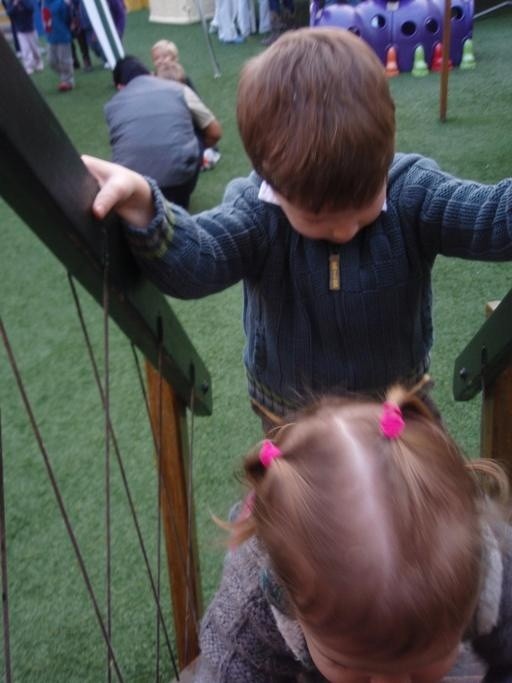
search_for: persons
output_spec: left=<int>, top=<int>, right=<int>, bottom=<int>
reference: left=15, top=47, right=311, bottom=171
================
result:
left=101, top=53, right=223, bottom=211
left=174, top=372, right=512, bottom=683
left=90, top=26, right=512, bottom=435
left=152, top=38, right=221, bottom=170
left=0, top=0, right=129, bottom=91
left=207, top=0, right=299, bottom=44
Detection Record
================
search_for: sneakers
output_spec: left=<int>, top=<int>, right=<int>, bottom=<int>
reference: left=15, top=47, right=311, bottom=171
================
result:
left=24, top=58, right=94, bottom=90
